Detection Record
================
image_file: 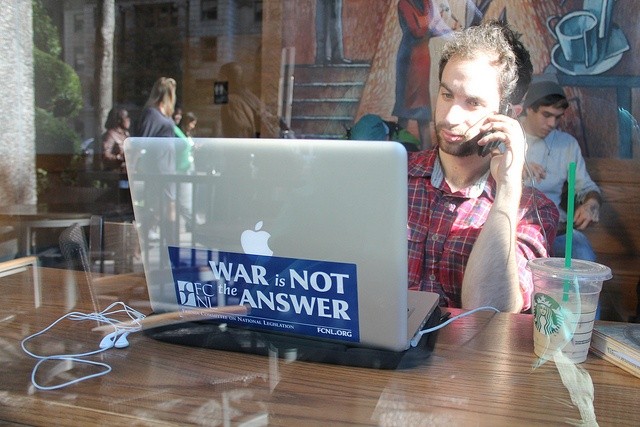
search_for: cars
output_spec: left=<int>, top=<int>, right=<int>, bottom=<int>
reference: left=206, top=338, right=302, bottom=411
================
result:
left=75, top=138, right=137, bottom=189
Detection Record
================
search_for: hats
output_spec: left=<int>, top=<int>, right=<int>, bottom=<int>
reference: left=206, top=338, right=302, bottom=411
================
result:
left=524, top=81, right=566, bottom=109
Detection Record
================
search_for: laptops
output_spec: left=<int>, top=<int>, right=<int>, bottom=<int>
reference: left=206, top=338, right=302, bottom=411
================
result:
left=124, top=136, right=440, bottom=351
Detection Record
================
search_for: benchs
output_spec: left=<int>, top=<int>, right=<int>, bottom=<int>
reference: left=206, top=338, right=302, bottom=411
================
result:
left=557, top=156, right=640, bottom=322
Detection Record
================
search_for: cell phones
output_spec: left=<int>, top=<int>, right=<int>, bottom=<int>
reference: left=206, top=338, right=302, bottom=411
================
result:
left=477, top=105, right=511, bottom=156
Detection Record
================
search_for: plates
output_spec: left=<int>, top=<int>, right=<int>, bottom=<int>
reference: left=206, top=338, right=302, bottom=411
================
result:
left=551, top=42, right=623, bottom=74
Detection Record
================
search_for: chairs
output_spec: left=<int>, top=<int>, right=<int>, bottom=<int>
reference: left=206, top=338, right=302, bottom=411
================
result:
left=58, top=222, right=248, bottom=358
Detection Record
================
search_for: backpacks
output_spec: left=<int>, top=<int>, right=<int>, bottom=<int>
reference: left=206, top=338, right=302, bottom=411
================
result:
left=230, top=91, right=296, bottom=138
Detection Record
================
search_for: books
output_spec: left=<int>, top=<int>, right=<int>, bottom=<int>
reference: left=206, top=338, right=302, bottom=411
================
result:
left=589, top=323, right=640, bottom=379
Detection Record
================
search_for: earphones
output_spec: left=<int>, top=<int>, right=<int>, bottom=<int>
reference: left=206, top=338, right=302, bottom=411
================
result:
left=99, top=329, right=124, bottom=349
left=114, top=331, right=130, bottom=348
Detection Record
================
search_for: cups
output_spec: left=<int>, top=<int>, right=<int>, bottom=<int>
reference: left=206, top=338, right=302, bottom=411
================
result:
left=546, top=11, right=598, bottom=62
left=526, top=258, right=615, bottom=366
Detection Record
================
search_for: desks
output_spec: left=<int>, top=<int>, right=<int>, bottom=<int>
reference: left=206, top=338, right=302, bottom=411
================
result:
left=1, top=211, right=91, bottom=257
left=0, top=263, right=640, bottom=426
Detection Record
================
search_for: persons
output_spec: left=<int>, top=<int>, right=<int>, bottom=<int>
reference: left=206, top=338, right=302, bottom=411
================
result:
left=100, top=107, right=143, bottom=189
left=218, top=61, right=295, bottom=138
left=498, top=81, right=603, bottom=320
left=406, top=19, right=561, bottom=314
left=180, top=109, right=196, bottom=137
left=134, top=74, right=177, bottom=247
left=171, top=105, right=197, bottom=232
